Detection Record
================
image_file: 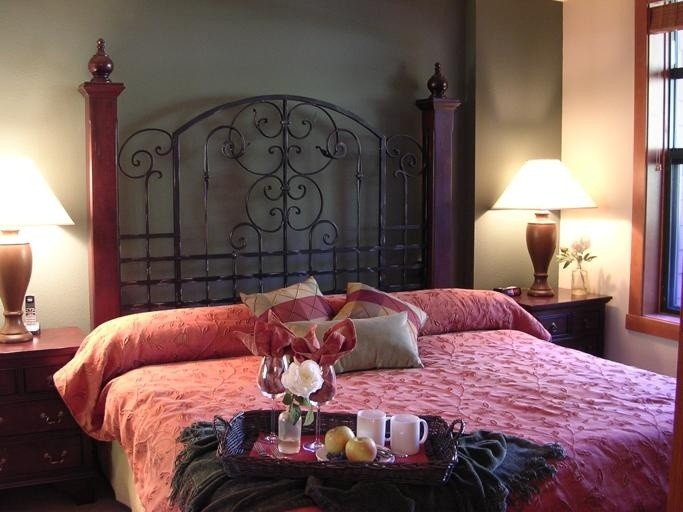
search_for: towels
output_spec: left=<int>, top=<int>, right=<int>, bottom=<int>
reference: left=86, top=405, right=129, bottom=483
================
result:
left=291, top=318, right=357, bottom=402
left=232, top=309, right=294, bottom=394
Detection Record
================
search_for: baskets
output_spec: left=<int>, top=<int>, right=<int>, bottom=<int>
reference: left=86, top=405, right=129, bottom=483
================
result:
left=212, top=411, right=466, bottom=487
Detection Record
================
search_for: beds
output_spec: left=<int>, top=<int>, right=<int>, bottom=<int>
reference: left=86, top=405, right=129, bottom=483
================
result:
left=50, top=44, right=676, bottom=511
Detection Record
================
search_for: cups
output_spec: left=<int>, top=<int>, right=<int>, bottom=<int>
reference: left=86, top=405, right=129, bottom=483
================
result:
left=389, top=413, right=429, bottom=455
left=356, top=410, right=390, bottom=449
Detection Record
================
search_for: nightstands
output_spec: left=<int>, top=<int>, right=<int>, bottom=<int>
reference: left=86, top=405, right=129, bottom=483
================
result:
left=501, top=287, right=612, bottom=359
left=0, top=325, right=95, bottom=512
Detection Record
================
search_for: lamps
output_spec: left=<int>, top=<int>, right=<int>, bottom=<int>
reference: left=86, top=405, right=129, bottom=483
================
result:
left=1, top=153, right=78, bottom=343
left=486, top=158, right=597, bottom=299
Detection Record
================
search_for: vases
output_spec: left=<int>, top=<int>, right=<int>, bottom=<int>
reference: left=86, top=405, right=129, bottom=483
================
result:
left=571, top=266, right=588, bottom=296
left=275, top=403, right=302, bottom=454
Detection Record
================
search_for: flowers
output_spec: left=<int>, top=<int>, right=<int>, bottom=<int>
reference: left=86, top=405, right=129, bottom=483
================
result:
left=550, top=239, right=599, bottom=270
left=280, top=359, right=325, bottom=427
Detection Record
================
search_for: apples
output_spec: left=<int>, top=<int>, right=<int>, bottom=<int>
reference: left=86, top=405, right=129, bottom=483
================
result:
left=346, top=437, right=378, bottom=463
left=325, top=425, right=354, bottom=454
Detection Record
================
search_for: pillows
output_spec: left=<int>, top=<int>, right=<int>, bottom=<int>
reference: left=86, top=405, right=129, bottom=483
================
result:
left=283, top=310, right=425, bottom=374
left=236, top=276, right=335, bottom=326
left=331, top=278, right=430, bottom=341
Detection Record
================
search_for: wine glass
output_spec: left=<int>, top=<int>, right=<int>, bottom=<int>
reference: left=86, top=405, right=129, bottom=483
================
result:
left=303, top=360, right=339, bottom=449
left=258, top=354, right=287, bottom=442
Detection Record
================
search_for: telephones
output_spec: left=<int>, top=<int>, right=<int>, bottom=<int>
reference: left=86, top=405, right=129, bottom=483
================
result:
left=23, top=296, right=40, bottom=335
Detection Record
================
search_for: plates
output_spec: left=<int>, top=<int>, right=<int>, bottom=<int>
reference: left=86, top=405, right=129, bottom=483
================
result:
left=315, top=442, right=394, bottom=465
